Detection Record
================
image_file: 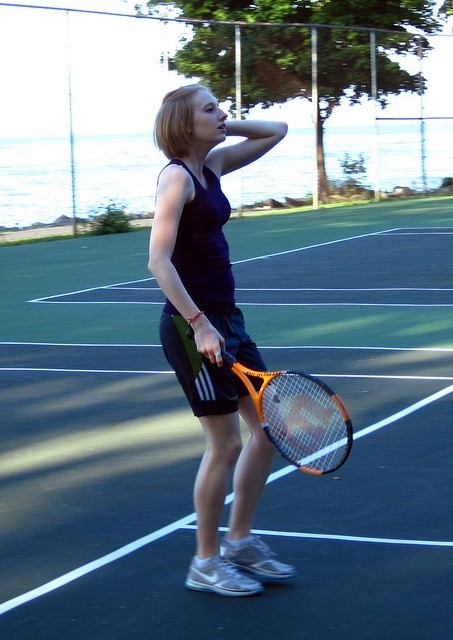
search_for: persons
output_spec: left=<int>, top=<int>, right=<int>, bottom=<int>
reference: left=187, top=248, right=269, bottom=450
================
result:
left=145, top=83, right=299, bottom=598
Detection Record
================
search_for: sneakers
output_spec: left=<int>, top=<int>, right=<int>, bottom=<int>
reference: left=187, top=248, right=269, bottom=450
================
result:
left=219, top=536, right=295, bottom=579
left=184, top=554, right=264, bottom=596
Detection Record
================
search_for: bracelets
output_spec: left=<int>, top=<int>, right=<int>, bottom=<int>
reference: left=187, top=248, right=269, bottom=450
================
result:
left=185, top=310, right=204, bottom=326
